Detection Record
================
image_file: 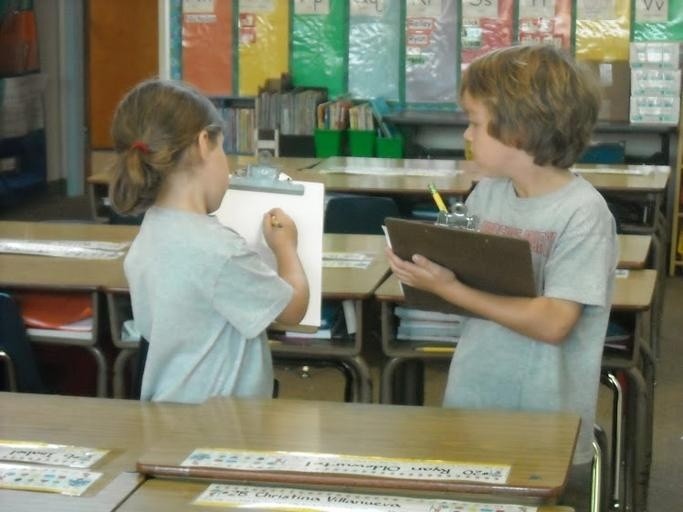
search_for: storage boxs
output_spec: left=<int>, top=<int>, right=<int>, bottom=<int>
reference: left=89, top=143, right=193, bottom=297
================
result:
left=313, top=124, right=405, bottom=160
left=575, top=41, right=682, bottom=123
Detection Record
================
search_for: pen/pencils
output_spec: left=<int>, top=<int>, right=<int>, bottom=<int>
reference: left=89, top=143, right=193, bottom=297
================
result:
left=269, top=215, right=282, bottom=232
left=428, top=184, right=450, bottom=214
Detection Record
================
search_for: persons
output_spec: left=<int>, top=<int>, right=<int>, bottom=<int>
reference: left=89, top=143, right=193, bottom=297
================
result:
left=382, top=41, right=620, bottom=510
left=102, top=79, right=309, bottom=405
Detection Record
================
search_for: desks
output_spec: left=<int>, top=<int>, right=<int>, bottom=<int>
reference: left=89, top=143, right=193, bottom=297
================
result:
left=0, top=151, right=675, bottom=510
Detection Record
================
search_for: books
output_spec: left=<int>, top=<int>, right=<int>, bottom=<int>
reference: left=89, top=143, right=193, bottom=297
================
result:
left=285, top=304, right=340, bottom=340
left=212, top=85, right=404, bottom=156
left=391, top=305, right=464, bottom=345
left=18, top=289, right=91, bottom=341
